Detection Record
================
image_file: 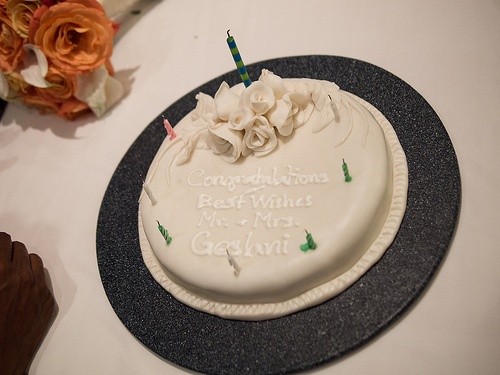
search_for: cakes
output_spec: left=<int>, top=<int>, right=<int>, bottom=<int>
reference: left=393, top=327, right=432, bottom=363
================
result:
left=137, top=67, right=410, bottom=322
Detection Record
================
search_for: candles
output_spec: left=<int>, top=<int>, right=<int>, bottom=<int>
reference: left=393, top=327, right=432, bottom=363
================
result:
left=304, top=229, right=316, bottom=249
left=156, top=220, right=172, bottom=244
left=328, top=94, right=340, bottom=122
left=142, top=180, right=157, bottom=206
left=162, top=115, right=176, bottom=141
left=227, top=29, right=252, bottom=88
left=226, top=249, right=241, bottom=274
left=342, top=159, right=352, bottom=182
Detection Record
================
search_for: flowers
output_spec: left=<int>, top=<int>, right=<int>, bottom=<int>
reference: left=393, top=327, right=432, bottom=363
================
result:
left=1, top=0, right=124, bottom=122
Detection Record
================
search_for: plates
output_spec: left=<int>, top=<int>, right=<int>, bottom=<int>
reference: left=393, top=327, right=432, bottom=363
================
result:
left=94, top=55, right=461, bottom=374
